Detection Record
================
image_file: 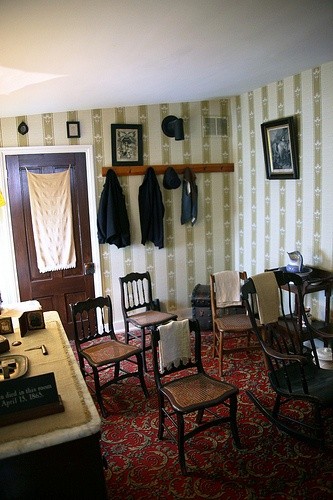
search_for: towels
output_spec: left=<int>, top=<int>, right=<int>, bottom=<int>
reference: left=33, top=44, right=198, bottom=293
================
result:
left=246, top=271, right=283, bottom=324
left=157, top=318, right=191, bottom=374
left=25, top=164, right=76, bottom=273
left=213, top=271, right=242, bottom=307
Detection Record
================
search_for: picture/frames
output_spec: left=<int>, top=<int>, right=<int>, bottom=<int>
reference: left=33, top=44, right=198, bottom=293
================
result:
left=260, top=116, right=300, bottom=180
left=111, top=124, right=144, bottom=166
left=67, top=121, right=81, bottom=139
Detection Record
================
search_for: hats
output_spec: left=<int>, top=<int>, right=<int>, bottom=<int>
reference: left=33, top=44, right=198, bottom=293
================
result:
left=163, top=167, right=181, bottom=191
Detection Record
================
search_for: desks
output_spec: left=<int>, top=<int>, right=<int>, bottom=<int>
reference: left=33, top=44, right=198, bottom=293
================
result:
left=0, top=311, right=108, bottom=500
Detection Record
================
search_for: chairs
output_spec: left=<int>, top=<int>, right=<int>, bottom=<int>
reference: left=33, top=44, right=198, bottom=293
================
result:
left=241, top=270, right=333, bottom=448
left=68, top=295, right=149, bottom=418
left=119, top=271, right=178, bottom=371
left=210, top=271, right=270, bottom=377
left=151, top=317, right=243, bottom=476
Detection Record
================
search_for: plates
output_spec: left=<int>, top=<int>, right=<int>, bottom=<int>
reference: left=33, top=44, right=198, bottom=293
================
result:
left=296, top=268, right=312, bottom=276
left=313, top=358, right=333, bottom=370
left=311, top=348, right=333, bottom=362
left=0, top=354, right=28, bottom=381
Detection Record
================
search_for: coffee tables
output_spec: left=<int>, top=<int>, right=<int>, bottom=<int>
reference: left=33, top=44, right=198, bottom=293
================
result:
left=265, top=265, right=333, bottom=348
